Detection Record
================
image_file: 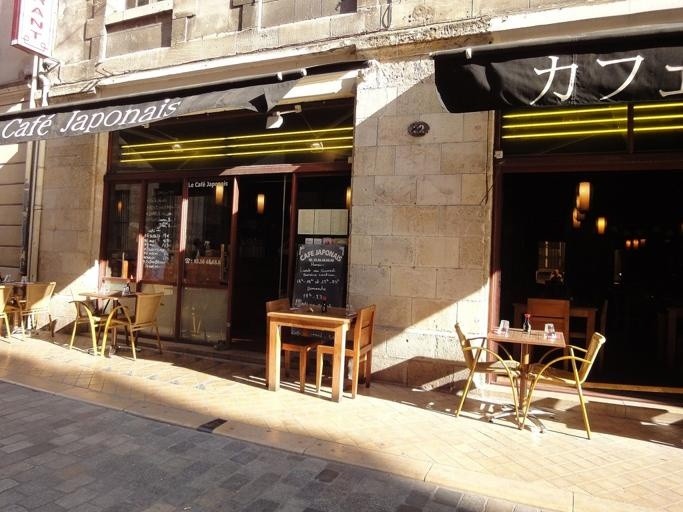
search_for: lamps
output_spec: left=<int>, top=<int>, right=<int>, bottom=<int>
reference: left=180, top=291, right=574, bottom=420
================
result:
left=68, top=285, right=129, bottom=355
left=316, top=304, right=376, bottom=398
left=265, top=297, right=326, bottom=393
left=570, top=300, right=609, bottom=368
left=455, top=321, right=522, bottom=428
left=0, top=279, right=56, bottom=343
left=102, top=293, right=165, bottom=360
left=521, top=297, right=571, bottom=370
left=520, top=331, right=606, bottom=440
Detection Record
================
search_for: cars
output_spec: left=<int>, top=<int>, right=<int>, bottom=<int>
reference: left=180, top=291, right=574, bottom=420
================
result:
left=346, top=303, right=353, bottom=318
left=295, top=298, right=303, bottom=308
left=498, top=320, right=511, bottom=337
left=21, top=276, right=27, bottom=284
left=5, top=273, right=12, bottom=283
left=11, top=294, right=27, bottom=305
left=544, top=323, right=557, bottom=339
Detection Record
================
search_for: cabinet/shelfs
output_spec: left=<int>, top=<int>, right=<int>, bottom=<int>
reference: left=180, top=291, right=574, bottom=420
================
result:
left=543, top=240, right=552, bottom=268
left=321, top=295, right=328, bottom=313
left=523, top=314, right=532, bottom=333
left=100, top=280, right=131, bottom=296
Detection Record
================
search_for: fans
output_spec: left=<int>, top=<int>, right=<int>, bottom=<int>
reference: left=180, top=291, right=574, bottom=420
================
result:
left=292, top=243, right=348, bottom=340
left=143, top=195, right=179, bottom=269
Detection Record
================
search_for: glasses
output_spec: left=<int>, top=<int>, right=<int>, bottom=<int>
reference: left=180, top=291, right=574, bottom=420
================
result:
left=571, top=167, right=608, bottom=235
left=265, top=104, right=302, bottom=129
